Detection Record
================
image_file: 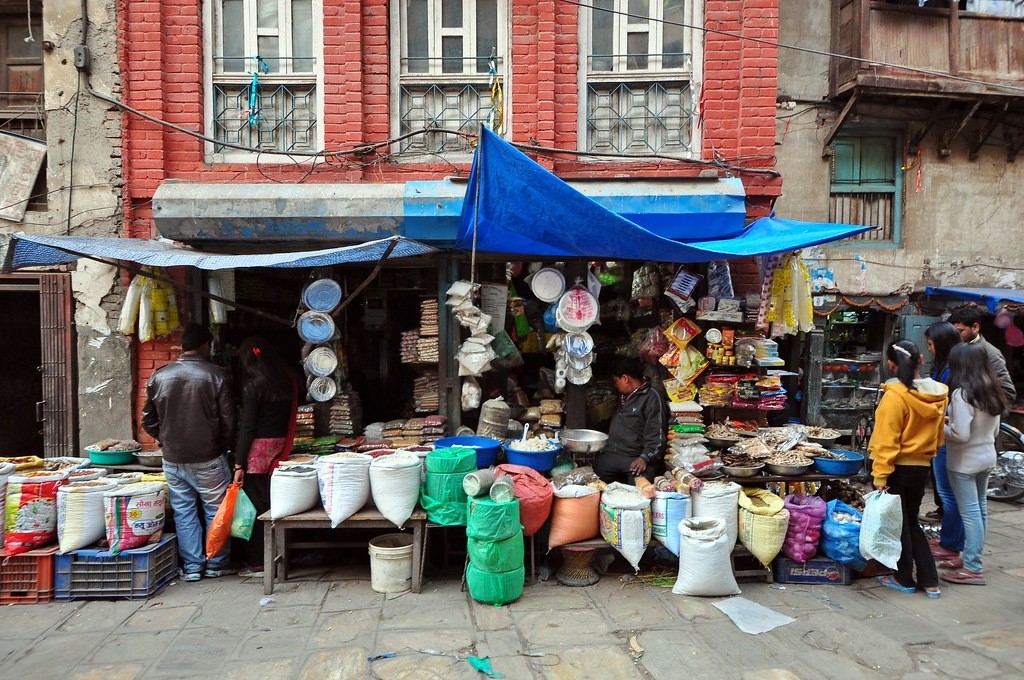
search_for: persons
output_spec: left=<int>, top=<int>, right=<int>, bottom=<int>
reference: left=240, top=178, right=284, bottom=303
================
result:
left=143, top=324, right=298, bottom=577
left=596, top=358, right=668, bottom=484
left=866, top=307, right=1016, bottom=598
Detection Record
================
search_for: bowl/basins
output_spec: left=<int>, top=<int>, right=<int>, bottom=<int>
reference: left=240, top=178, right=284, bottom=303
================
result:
left=84, top=445, right=143, bottom=464
left=132, top=450, right=163, bottom=466
left=707, top=427, right=865, bottom=475
left=503, top=438, right=560, bottom=471
left=559, top=428, right=610, bottom=453
left=433, top=436, right=501, bottom=470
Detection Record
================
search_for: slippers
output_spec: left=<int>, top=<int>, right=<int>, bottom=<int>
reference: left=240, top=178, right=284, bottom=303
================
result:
left=918, top=512, right=943, bottom=521
left=941, top=570, right=986, bottom=585
left=939, top=559, right=964, bottom=569
left=916, top=580, right=942, bottom=599
left=930, top=539, right=960, bottom=559
left=238, top=564, right=264, bottom=577
left=877, top=576, right=917, bottom=593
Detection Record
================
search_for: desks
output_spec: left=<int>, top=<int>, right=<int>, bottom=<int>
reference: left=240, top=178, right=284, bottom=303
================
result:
left=257, top=508, right=425, bottom=594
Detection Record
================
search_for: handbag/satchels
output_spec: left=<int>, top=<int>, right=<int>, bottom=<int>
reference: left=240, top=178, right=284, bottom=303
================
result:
left=206, top=483, right=240, bottom=560
left=858, top=489, right=903, bottom=571
left=230, top=487, right=257, bottom=542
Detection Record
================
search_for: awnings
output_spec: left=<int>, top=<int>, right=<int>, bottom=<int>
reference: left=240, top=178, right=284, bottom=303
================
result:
left=451, top=123, right=877, bottom=266
left=0, top=230, right=448, bottom=274
left=926, top=285, right=1024, bottom=312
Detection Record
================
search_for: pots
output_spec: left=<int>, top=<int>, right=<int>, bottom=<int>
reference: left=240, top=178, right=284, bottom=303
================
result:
left=307, top=347, right=337, bottom=377
left=556, top=283, right=597, bottom=384
left=297, top=311, right=335, bottom=344
left=310, top=378, right=336, bottom=402
left=532, top=268, right=565, bottom=303
left=305, top=279, right=341, bottom=313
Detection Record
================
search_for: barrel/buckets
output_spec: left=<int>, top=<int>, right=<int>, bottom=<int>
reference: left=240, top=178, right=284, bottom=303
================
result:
left=367, top=533, right=414, bottom=592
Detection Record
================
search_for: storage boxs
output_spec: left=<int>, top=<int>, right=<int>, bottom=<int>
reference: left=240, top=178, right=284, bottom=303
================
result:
left=774, top=558, right=850, bottom=588
left=0, top=532, right=181, bottom=604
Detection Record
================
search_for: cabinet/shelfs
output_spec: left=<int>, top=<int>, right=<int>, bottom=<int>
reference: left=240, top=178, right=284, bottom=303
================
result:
left=822, top=354, right=882, bottom=437
left=699, top=317, right=771, bottom=427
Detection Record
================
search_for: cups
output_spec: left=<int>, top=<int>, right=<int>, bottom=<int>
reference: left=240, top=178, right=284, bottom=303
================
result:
left=120, top=282, right=153, bottom=340
left=209, top=278, right=227, bottom=324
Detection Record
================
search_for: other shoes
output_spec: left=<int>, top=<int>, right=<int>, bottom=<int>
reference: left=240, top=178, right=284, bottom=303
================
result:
left=205, top=569, right=223, bottom=577
left=185, top=572, right=201, bottom=582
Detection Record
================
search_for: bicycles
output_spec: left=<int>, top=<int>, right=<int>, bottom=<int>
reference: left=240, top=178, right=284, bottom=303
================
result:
left=852, top=386, right=1024, bottom=502
